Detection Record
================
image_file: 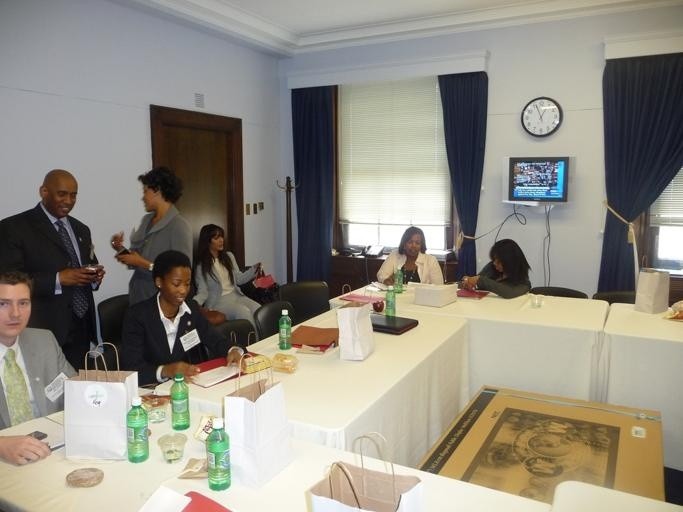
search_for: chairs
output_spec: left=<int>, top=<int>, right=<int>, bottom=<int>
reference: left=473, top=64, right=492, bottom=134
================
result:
left=97, top=295, right=130, bottom=353
left=279, top=281, right=329, bottom=325
left=207, top=319, right=255, bottom=358
left=254, top=301, right=295, bottom=340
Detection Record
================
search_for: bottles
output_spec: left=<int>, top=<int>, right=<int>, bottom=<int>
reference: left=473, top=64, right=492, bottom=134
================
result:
left=279, top=310, right=292, bottom=350
left=126, top=397, right=149, bottom=462
left=170, top=374, right=190, bottom=430
left=207, top=418, right=231, bottom=490
left=394, top=266, right=403, bottom=293
left=386, top=286, right=395, bottom=316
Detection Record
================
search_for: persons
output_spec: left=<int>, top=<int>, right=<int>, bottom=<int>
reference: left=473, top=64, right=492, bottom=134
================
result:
left=461, top=239, right=532, bottom=300
left=0, top=168, right=106, bottom=371
left=110, top=165, right=195, bottom=306
left=377, top=227, right=444, bottom=285
left=0, top=270, right=79, bottom=468
left=192, top=224, right=264, bottom=342
left=119, top=249, right=248, bottom=388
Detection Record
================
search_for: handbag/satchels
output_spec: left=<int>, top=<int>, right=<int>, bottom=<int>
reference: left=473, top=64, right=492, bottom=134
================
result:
left=308, top=430, right=420, bottom=512
left=634, top=255, right=670, bottom=313
left=223, top=354, right=283, bottom=463
left=63, top=341, right=139, bottom=461
left=338, top=284, right=375, bottom=362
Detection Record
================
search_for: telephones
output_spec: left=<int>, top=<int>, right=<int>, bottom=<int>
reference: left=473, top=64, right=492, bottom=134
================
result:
left=361, top=244, right=385, bottom=258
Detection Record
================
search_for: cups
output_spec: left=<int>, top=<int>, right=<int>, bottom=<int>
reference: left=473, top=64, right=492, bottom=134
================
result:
left=158, top=433, right=188, bottom=465
left=530, top=295, right=543, bottom=308
left=144, top=398, right=168, bottom=424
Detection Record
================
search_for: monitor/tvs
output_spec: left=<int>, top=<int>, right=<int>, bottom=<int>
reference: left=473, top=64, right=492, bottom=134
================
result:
left=509, top=157, right=570, bottom=202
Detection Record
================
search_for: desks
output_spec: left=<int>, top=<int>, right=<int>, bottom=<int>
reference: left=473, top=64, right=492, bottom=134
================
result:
left=153, top=302, right=470, bottom=468
left=598, top=301, right=683, bottom=474
left=329, top=279, right=608, bottom=403
left=0, top=407, right=550, bottom=512
left=552, top=479, right=683, bottom=512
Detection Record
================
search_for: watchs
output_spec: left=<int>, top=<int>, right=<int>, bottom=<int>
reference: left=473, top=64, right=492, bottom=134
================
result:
left=148, top=262, right=154, bottom=272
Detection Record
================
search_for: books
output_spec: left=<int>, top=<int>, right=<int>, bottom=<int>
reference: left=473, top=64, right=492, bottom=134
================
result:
left=369, top=312, right=419, bottom=335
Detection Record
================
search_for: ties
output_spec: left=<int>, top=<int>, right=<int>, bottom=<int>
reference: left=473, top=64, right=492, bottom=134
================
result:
left=2, top=349, right=36, bottom=426
left=55, top=220, right=90, bottom=319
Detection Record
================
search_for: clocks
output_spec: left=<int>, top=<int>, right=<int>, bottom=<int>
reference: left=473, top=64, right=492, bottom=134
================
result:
left=521, top=96, right=563, bottom=138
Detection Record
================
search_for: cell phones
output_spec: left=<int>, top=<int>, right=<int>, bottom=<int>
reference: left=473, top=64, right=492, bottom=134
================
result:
left=115, top=249, right=132, bottom=258
